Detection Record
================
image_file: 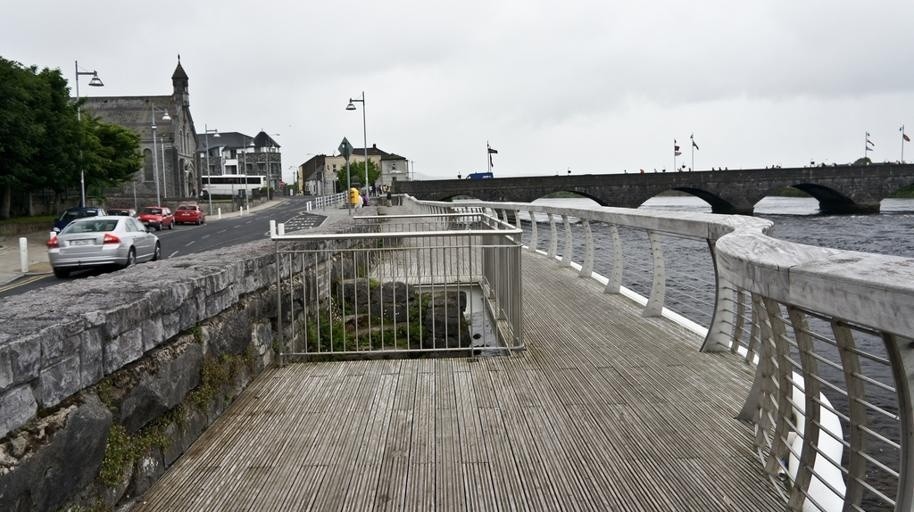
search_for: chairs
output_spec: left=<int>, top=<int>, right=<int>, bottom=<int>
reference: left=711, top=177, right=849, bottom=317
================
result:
left=105, top=224, right=114, bottom=230
left=81, top=225, right=97, bottom=232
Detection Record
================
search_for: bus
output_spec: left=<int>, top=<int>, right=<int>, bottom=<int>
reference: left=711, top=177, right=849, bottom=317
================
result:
left=201, top=175, right=267, bottom=199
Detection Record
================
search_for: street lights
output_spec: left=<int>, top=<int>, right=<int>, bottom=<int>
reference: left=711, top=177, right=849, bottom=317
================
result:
left=346, top=91, right=370, bottom=206
left=205, top=124, right=220, bottom=216
left=244, top=138, right=256, bottom=210
left=265, top=143, right=276, bottom=201
left=75, top=60, right=103, bottom=208
left=151, top=102, right=171, bottom=207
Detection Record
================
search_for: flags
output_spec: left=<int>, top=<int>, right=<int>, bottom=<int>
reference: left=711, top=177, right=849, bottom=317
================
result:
left=693, top=141, right=699, bottom=148
left=867, top=140, right=875, bottom=145
left=866, top=147, right=874, bottom=151
left=488, top=148, right=497, bottom=154
left=903, top=133, right=910, bottom=142
left=675, top=145, right=680, bottom=155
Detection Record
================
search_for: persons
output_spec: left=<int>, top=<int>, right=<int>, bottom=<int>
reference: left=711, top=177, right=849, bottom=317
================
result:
left=356, top=184, right=392, bottom=209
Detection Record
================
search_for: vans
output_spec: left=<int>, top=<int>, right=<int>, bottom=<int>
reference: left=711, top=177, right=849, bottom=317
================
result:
left=466, top=173, right=493, bottom=179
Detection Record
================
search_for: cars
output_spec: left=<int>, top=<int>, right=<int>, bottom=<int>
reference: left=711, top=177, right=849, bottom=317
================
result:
left=47, top=207, right=162, bottom=279
left=175, top=203, right=205, bottom=224
left=136, top=206, right=175, bottom=230
